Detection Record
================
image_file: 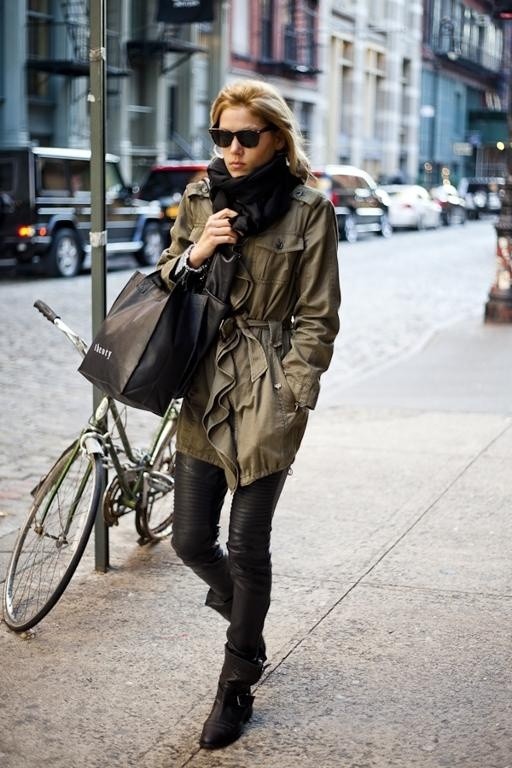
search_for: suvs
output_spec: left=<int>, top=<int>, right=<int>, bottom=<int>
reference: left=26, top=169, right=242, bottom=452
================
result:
left=0, top=145, right=168, bottom=276
left=309, top=166, right=395, bottom=240
left=138, top=158, right=211, bottom=243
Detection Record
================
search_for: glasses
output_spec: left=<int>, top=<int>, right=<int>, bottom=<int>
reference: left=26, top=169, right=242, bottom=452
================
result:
left=209, top=126, right=273, bottom=149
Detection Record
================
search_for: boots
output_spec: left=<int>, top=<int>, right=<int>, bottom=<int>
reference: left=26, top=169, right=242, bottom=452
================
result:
left=203, top=585, right=235, bottom=626
left=198, top=642, right=271, bottom=751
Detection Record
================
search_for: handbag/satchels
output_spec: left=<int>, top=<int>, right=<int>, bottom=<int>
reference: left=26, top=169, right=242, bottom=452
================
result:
left=77, top=269, right=226, bottom=420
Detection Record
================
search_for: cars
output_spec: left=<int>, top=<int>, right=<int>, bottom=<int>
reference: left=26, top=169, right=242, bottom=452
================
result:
left=379, top=183, right=443, bottom=229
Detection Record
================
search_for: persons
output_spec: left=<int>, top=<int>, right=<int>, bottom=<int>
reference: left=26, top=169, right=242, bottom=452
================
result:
left=155, top=78, right=341, bottom=749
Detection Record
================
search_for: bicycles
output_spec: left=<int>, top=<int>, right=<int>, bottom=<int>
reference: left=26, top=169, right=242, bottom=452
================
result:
left=6, top=298, right=181, bottom=629
